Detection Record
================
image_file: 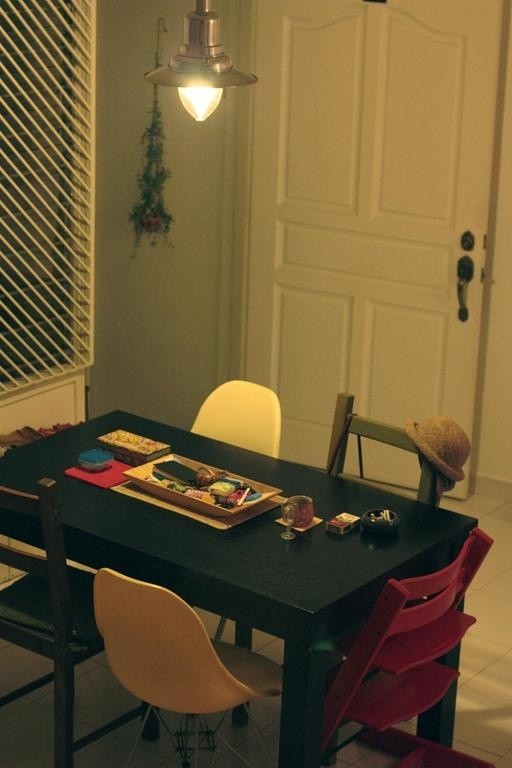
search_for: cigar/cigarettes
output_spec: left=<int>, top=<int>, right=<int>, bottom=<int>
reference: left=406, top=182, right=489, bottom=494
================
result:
left=370, top=509, right=390, bottom=523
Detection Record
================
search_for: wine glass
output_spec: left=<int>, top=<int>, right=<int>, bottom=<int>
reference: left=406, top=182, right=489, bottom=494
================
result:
left=280, top=502, right=296, bottom=541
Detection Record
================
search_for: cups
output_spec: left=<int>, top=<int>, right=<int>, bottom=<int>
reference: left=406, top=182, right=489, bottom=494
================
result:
left=289, top=495, right=314, bottom=526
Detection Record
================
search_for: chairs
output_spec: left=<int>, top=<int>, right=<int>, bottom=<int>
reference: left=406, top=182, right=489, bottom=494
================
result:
left=90, top=568, right=281, bottom=767
left=0, top=487, right=160, bottom=767
left=190, top=380, right=283, bottom=461
left=325, top=392, right=471, bottom=506
left=317, top=527, right=493, bottom=763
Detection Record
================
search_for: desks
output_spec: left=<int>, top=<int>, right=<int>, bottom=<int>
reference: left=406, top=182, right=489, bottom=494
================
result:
left=5, top=411, right=481, bottom=766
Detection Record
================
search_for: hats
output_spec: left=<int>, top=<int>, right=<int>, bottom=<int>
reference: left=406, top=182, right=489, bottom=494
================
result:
left=405, top=415, right=471, bottom=482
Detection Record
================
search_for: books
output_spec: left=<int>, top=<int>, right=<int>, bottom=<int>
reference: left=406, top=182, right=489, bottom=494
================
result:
left=152, top=460, right=198, bottom=488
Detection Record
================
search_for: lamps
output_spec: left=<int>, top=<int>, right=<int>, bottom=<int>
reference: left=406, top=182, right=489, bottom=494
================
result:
left=142, top=1, right=259, bottom=123
